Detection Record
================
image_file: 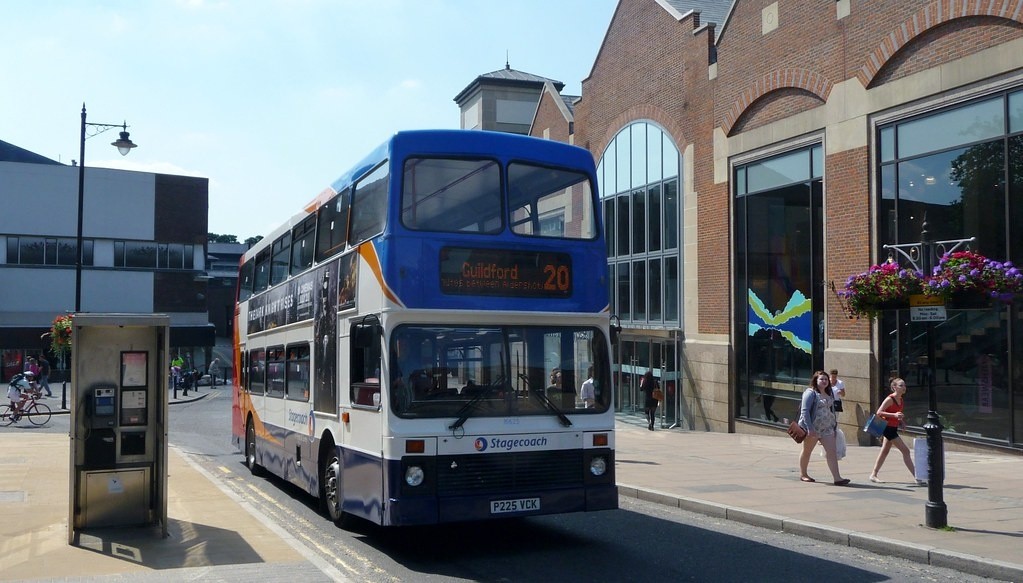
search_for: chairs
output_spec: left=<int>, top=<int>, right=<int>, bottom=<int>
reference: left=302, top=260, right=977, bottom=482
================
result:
left=244, top=368, right=516, bottom=417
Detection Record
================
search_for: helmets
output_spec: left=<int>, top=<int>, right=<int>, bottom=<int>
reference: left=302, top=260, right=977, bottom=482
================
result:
left=24, top=371, right=34, bottom=376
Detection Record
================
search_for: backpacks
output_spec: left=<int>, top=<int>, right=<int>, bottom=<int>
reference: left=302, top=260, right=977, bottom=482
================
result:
left=8, top=374, right=23, bottom=386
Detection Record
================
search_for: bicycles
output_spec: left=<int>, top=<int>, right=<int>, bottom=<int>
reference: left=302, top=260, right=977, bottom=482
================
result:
left=0, top=393, right=52, bottom=427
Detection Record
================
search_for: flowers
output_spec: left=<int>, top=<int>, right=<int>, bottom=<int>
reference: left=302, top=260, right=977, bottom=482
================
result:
left=839, top=250, right=1023, bottom=320
left=49, top=313, right=72, bottom=357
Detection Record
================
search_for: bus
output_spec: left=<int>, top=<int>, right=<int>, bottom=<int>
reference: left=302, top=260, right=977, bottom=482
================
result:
left=232, top=128, right=623, bottom=527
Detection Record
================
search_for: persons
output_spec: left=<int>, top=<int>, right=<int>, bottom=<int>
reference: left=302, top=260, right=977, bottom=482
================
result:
left=797, top=371, right=850, bottom=485
left=639, top=372, right=660, bottom=431
left=374, top=335, right=426, bottom=411
left=581, top=362, right=595, bottom=408
left=870, top=377, right=927, bottom=486
left=208, top=358, right=222, bottom=389
left=8, top=355, right=52, bottom=424
left=549, top=368, right=563, bottom=408
left=756, top=372, right=779, bottom=423
left=818, top=368, right=845, bottom=445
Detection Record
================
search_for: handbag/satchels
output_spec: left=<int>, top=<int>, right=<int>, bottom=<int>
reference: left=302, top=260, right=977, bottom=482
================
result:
left=830, top=399, right=843, bottom=412
left=787, top=421, right=807, bottom=444
left=820, top=429, right=846, bottom=461
left=652, top=380, right=664, bottom=401
left=862, top=413, right=888, bottom=437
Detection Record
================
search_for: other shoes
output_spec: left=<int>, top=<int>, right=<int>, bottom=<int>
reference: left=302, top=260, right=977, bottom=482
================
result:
left=9, top=415, right=17, bottom=423
left=648, top=423, right=654, bottom=431
left=834, top=478, right=850, bottom=486
left=211, top=386, right=216, bottom=389
left=19, top=409, right=25, bottom=412
left=45, top=394, right=51, bottom=396
left=915, top=478, right=928, bottom=487
left=765, top=415, right=779, bottom=422
left=870, top=475, right=880, bottom=483
left=800, top=474, right=815, bottom=482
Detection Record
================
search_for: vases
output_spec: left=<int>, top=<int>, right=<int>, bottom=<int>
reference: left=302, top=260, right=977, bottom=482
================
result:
left=882, top=298, right=910, bottom=310
left=950, top=287, right=971, bottom=305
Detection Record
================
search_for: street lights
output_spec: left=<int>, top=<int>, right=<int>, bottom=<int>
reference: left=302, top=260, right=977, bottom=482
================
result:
left=74, top=121, right=139, bottom=313
